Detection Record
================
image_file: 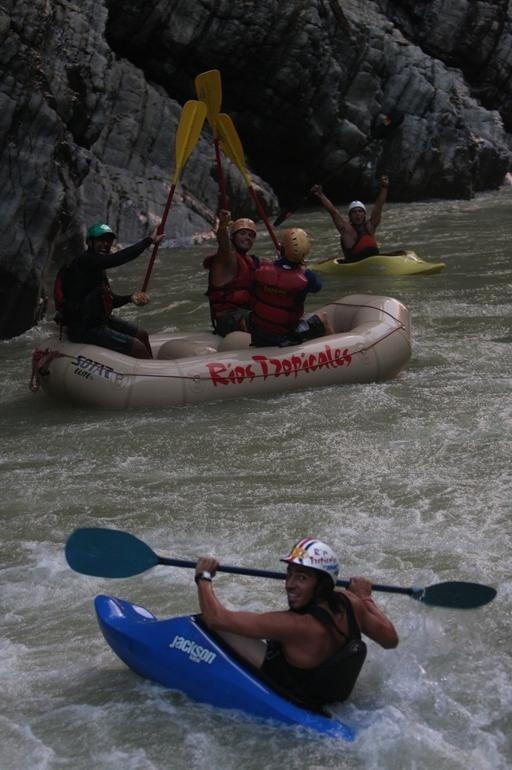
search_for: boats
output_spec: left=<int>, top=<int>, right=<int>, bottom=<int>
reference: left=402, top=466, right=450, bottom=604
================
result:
left=23, top=289, right=416, bottom=414
left=92, top=592, right=360, bottom=746
left=295, top=245, right=445, bottom=279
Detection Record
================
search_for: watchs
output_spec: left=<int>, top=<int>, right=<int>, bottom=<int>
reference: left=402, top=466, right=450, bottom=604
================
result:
left=194, top=570, right=213, bottom=585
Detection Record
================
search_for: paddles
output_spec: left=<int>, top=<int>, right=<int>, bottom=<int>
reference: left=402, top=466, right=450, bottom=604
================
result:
left=143, top=99, right=208, bottom=292
left=216, top=113, right=279, bottom=249
left=195, top=69, right=227, bottom=210
left=65, top=528, right=498, bottom=609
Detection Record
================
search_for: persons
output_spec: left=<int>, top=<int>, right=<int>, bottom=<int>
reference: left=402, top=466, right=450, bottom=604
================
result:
left=199, top=206, right=272, bottom=337
left=310, top=175, right=391, bottom=263
left=51, top=221, right=166, bottom=359
left=247, top=227, right=334, bottom=348
left=191, top=536, right=399, bottom=711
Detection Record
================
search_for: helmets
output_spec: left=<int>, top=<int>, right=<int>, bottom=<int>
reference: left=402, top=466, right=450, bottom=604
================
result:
left=231, top=218, right=257, bottom=236
left=282, top=227, right=309, bottom=264
left=348, top=200, right=367, bottom=214
left=86, top=222, right=118, bottom=244
left=280, top=538, right=342, bottom=586
